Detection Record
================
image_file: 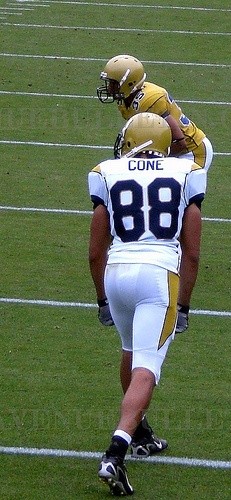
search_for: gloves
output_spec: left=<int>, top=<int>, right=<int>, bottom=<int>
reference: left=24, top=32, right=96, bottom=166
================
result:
left=174, top=303, right=189, bottom=333
left=96, top=298, right=115, bottom=327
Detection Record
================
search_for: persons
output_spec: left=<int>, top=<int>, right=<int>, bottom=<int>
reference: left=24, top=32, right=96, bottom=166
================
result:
left=97, top=55, right=213, bottom=171
left=88, top=112, right=207, bottom=497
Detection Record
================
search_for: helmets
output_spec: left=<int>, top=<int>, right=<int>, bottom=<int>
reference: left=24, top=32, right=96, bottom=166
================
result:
left=97, top=54, right=144, bottom=103
left=114, top=112, right=172, bottom=158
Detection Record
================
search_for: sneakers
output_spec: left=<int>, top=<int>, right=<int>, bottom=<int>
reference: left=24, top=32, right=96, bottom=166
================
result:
left=98, top=453, right=133, bottom=496
left=131, top=417, right=168, bottom=458
